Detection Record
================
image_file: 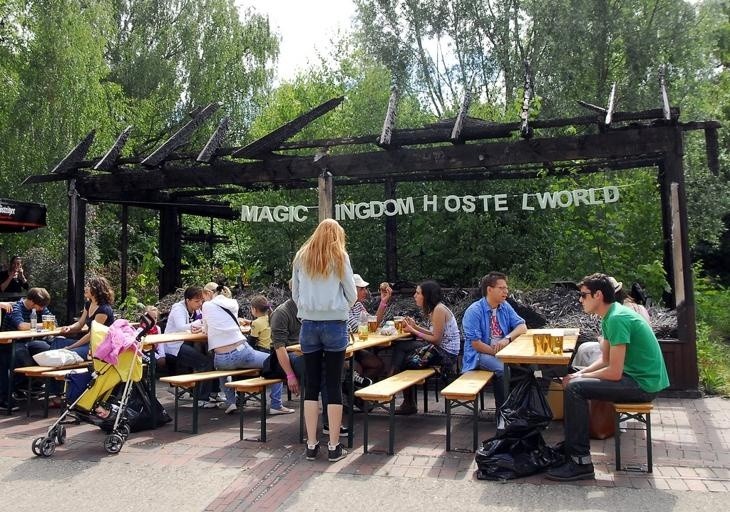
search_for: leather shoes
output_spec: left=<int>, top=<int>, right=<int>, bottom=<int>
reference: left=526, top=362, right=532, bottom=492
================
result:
left=546, top=461, right=595, bottom=482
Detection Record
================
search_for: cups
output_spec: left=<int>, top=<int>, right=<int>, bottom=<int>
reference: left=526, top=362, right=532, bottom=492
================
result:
left=530, top=329, right=564, bottom=356
left=42, top=315, right=55, bottom=330
left=393, top=315, right=403, bottom=335
left=366, top=315, right=378, bottom=332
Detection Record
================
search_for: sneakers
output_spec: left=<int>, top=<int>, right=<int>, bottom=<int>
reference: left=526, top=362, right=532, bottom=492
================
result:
left=323, top=425, right=349, bottom=437
left=225, top=404, right=237, bottom=414
left=306, top=440, right=321, bottom=461
left=198, top=394, right=227, bottom=408
left=353, top=370, right=372, bottom=389
left=270, top=408, right=295, bottom=415
left=328, top=441, right=347, bottom=462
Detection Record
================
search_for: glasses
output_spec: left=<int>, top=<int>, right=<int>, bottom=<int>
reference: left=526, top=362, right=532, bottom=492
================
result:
left=579, top=292, right=591, bottom=298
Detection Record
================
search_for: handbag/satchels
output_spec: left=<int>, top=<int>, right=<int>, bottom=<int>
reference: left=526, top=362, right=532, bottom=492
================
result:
left=32, top=349, right=84, bottom=367
left=405, top=344, right=439, bottom=369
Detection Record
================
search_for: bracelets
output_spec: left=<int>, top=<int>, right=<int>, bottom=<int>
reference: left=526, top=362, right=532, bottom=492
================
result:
left=419, top=328, right=425, bottom=333
left=379, top=302, right=388, bottom=309
left=417, top=332, right=424, bottom=338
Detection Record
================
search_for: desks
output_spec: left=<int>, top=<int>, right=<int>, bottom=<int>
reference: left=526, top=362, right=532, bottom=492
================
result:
left=1, top=322, right=140, bottom=413
left=141, top=325, right=251, bottom=397
left=288, top=328, right=413, bottom=447
left=496, top=326, right=579, bottom=442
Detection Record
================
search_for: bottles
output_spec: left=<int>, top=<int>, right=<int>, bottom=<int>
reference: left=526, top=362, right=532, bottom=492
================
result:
left=29, top=308, right=37, bottom=331
left=356, top=309, right=370, bottom=341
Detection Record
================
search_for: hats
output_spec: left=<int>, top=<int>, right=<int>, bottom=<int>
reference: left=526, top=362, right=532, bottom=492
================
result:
left=353, top=274, right=369, bottom=288
left=608, top=277, right=623, bottom=293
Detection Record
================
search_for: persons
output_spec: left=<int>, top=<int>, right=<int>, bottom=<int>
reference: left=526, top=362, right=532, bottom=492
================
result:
left=163, top=283, right=295, bottom=415
left=343, top=274, right=393, bottom=377
left=259, top=278, right=373, bottom=437
left=139, top=305, right=166, bottom=364
left=394, top=280, right=461, bottom=415
left=544, top=272, right=671, bottom=482
left=0, top=255, right=56, bottom=412
left=48, top=276, right=114, bottom=408
left=460, top=271, right=527, bottom=417
left=570, top=276, right=651, bottom=440
left=292, top=219, right=359, bottom=462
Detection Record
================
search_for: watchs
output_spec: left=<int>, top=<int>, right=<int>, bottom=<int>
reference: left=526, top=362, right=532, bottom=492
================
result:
left=505, top=335, right=511, bottom=344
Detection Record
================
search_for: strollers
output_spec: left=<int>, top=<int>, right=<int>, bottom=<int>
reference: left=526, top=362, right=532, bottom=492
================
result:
left=29, top=311, right=160, bottom=459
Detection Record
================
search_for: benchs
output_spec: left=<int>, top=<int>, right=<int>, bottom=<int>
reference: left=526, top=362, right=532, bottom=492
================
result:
left=159, top=369, right=291, bottom=433
left=13, top=359, right=147, bottom=420
left=614, top=402, right=655, bottom=473
left=225, top=378, right=284, bottom=444
left=440, top=370, right=493, bottom=455
left=355, top=364, right=441, bottom=456
